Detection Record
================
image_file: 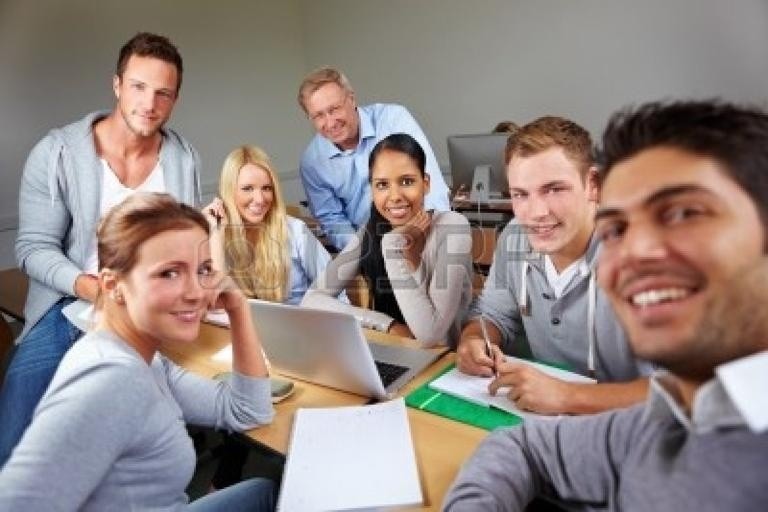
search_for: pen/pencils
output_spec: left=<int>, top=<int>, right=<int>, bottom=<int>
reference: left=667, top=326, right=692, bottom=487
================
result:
left=478, top=317, right=501, bottom=380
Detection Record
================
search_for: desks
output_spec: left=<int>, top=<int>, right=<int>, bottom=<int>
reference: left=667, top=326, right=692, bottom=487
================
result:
left=0, top=194, right=598, bottom=512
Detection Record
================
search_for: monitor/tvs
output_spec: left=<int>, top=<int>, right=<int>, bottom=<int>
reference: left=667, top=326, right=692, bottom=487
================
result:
left=447, top=133, right=513, bottom=203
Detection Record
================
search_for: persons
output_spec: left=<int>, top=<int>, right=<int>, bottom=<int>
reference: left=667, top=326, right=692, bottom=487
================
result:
left=0, top=31, right=766, bottom=508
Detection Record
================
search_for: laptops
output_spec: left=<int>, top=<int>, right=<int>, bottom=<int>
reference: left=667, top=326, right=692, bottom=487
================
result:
left=248, top=298, right=439, bottom=400
